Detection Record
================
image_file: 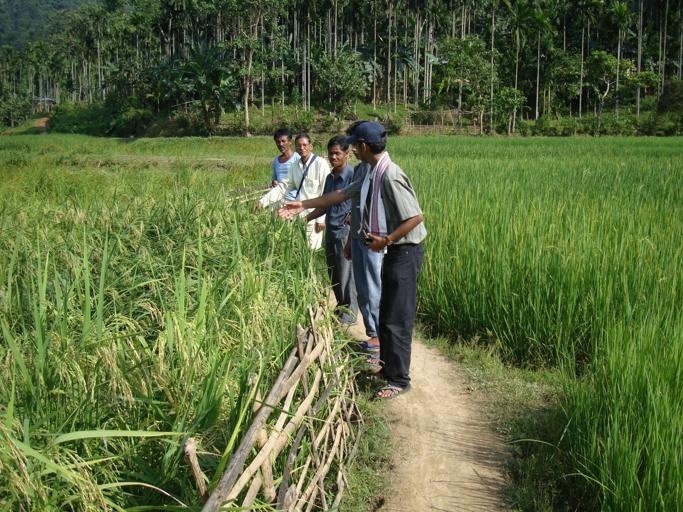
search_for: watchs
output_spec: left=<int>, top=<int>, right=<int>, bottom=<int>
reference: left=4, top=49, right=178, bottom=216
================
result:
left=384, top=235, right=394, bottom=246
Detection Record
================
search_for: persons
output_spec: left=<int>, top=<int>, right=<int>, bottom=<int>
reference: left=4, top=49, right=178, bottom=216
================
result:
left=322, top=134, right=359, bottom=327
left=277, top=121, right=428, bottom=400
left=272, top=128, right=301, bottom=201
left=252, top=133, right=331, bottom=250
left=344, top=121, right=388, bottom=367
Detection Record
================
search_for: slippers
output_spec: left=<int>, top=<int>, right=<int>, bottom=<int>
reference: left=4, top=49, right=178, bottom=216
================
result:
left=356, top=338, right=380, bottom=350
left=368, top=372, right=410, bottom=400
left=365, top=352, right=383, bottom=367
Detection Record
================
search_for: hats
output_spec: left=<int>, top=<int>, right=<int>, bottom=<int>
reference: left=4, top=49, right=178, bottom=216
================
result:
left=344, top=120, right=386, bottom=145
